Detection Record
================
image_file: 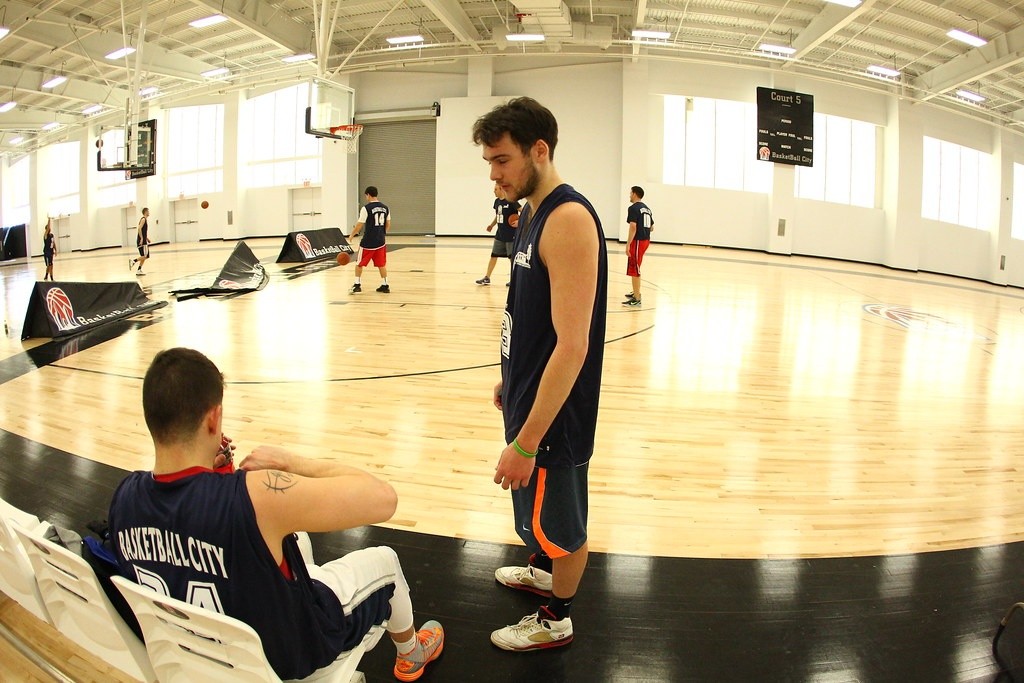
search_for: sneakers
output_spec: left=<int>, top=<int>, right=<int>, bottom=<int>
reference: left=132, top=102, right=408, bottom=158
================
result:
left=506, top=281, right=511, bottom=286
left=128, top=258, right=135, bottom=271
left=622, top=298, right=642, bottom=307
left=135, top=269, right=146, bottom=275
left=376, top=284, right=390, bottom=293
left=494, top=564, right=553, bottom=598
left=393, top=620, right=445, bottom=682
left=625, top=291, right=636, bottom=298
left=349, top=284, right=361, bottom=294
left=476, top=276, right=491, bottom=284
left=490, top=605, right=573, bottom=652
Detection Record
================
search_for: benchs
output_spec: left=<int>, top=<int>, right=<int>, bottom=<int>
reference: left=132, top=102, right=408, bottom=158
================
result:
left=1, top=498, right=386, bottom=683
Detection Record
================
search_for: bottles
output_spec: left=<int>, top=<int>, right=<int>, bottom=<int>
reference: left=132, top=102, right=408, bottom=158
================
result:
left=304, top=179, right=310, bottom=186
left=214, top=436, right=236, bottom=474
left=4, top=320, right=9, bottom=335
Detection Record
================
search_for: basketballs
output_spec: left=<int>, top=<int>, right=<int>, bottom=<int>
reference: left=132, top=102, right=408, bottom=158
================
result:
left=96, top=140, right=103, bottom=148
left=336, top=252, right=350, bottom=265
left=201, top=201, right=208, bottom=209
left=508, top=214, right=520, bottom=227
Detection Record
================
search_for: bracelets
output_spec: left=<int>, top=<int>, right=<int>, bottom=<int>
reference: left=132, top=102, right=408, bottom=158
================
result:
left=513, top=439, right=538, bottom=457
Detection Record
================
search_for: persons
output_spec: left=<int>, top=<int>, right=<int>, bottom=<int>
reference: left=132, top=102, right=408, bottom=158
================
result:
left=622, top=186, right=654, bottom=307
left=129, top=208, right=151, bottom=275
left=107, top=347, right=443, bottom=681
left=476, top=184, right=522, bottom=286
left=43, top=218, right=58, bottom=280
left=471, top=97, right=608, bottom=651
left=348, top=186, right=390, bottom=294
